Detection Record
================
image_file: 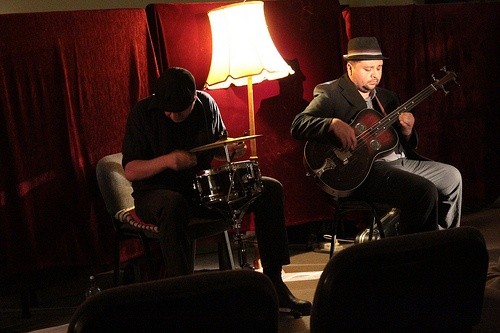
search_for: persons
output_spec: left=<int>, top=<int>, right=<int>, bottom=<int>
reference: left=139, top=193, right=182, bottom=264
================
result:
left=122, top=66, right=312, bottom=312
left=290, top=36, right=463, bottom=236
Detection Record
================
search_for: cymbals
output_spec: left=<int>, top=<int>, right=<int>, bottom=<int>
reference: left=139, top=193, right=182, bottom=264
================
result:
left=188, top=133, right=263, bottom=153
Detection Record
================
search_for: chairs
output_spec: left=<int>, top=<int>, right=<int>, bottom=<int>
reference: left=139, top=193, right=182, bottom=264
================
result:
left=68, top=269, right=279, bottom=333
left=327, top=195, right=394, bottom=259
left=96, top=151, right=234, bottom=283
left=309, top=227, right=489, bottom=333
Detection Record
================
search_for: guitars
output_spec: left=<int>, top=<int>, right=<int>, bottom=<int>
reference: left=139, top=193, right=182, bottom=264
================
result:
left=301, top=64, right=462, bottom=199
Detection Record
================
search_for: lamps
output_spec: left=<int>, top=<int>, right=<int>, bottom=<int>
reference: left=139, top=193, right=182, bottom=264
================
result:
left=204, top=2, right=296, bottom=269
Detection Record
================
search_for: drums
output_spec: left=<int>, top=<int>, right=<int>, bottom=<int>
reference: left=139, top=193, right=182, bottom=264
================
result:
left=193, top=156, right=265, bottom=206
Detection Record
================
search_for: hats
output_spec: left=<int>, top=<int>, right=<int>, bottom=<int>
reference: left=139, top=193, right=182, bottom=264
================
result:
left=155, top=66, right=196, bottom=113
left=343, top=37, right=388, bottom=60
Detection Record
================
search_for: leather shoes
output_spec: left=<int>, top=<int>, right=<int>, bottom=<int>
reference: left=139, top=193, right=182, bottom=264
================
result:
left=274, top=282, right=312, bottom=316
